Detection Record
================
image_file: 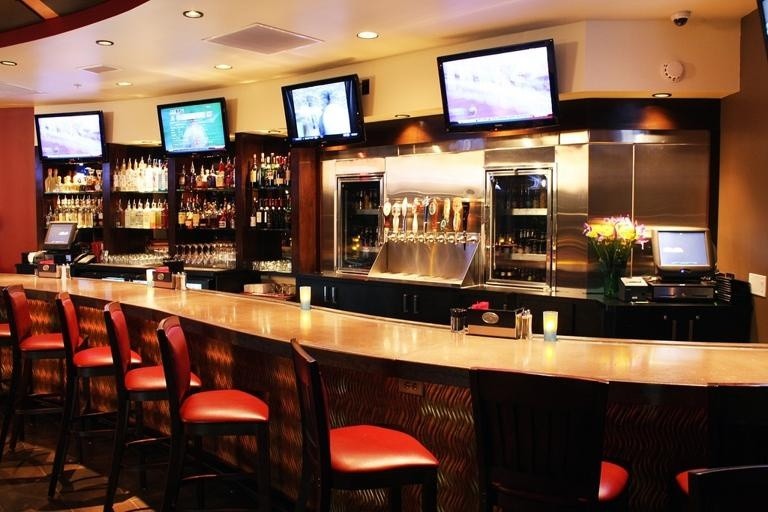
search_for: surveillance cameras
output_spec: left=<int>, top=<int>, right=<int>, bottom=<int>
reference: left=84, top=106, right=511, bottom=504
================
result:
left=670, top=10, right=691, bottom=27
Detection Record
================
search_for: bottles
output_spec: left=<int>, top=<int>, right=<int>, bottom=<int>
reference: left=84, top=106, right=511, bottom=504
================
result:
left=45, top=195, right=103, bottom=227
left=178, top=193, right=234, bottom=229
left=45, top=168, right=103, bottom=192
left=357, top=223, right=377, bottom=247
left=501, top=183, right=547, bottom=209
left=250, top=194, right=291, bottom=228
left=114, top=198, right=168, bottom=229
left=494, top=266, right=545, bottom=282
left=354, top=189, right=378, bottom=209
left=249, top=152, right=291, bottom=187
left=499, top=227, right=546, bottom=254
left=175, top=272, right=186, bottom=290
left=61, top=264, right=71, bottom=279
left=179, top=156, right=235, bottom=188
left=113, top=155, right=168, bottom=192
left=521, top=311, right=532, bottom=339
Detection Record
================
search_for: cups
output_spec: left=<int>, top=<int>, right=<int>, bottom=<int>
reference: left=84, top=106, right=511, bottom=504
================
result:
left=146, top=269, right=156, bottom=286
left=299, top=285, right=312, bottom=309
left=104, top=254, right=154, bottom=265
left=252, top=259, right=292, bottom=273
left=543, top=311, right=558, bottom=341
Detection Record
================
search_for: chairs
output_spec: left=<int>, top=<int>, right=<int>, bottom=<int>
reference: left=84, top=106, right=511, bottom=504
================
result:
left=156, top=315, right=272, bottom=511
left=1, top=324, right=12, bottom=342
left=673, top=465, right=768, bottom=512
left=3, top=284, right=91, bottom=462
left=47, top=291, right=146, bottom=502
left=470, top=366, right=631, bottom=512
left=104, top=300, right=206, bottom=510
left=291, top=338, right=442, bottom=511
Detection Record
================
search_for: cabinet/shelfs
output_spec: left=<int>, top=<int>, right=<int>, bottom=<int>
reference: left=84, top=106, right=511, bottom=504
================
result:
left=493, top=205, right=548, bottom=264
left=350, top=208, right=380, bottom=253
left=16, top=137, right=321, bottom=277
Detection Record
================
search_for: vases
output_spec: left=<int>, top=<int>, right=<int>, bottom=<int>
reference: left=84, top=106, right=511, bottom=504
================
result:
left=599, top=261, right=625, bottom=298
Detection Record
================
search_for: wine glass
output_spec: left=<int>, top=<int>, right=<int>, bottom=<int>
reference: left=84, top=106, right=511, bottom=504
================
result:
left=204, top=243, right=236, bottom=269
left=172, top=244, right=204, bottom=264
left=154, top=250, right=169, bottom=265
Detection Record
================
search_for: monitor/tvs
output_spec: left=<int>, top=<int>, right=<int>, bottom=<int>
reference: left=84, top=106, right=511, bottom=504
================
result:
left=281, top=73, right=364, bottom=147
left=436, top=38, right=561, bottom=133
left=43, top=222, right=78, bottom=250
left=35, top=110, right=109, bottom=165
left=156, top=96, right=231, bottom=157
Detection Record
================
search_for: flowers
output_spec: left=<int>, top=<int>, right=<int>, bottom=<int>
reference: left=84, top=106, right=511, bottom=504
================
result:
left=579, top=214, right=653, bottom=262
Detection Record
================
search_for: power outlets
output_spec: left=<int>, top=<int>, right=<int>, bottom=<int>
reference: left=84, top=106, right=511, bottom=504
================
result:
left=398, top=376, right=424, bottom=396
